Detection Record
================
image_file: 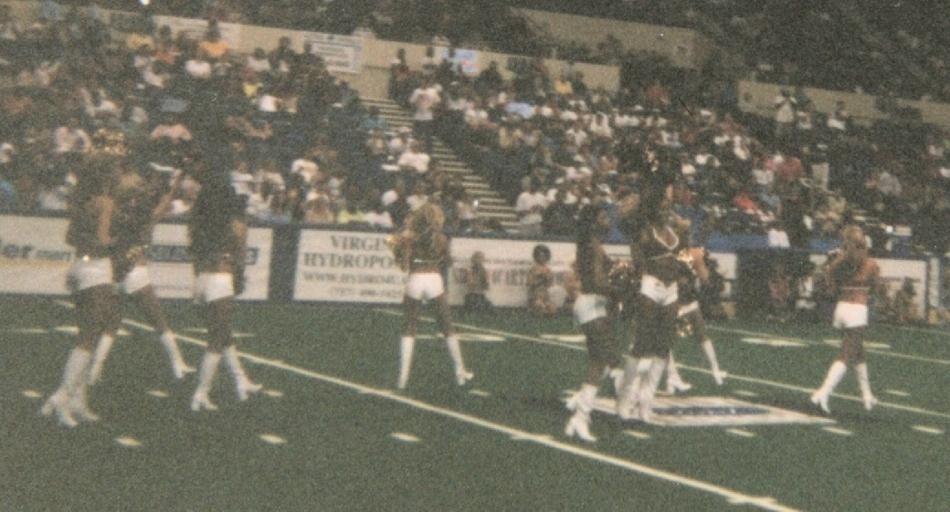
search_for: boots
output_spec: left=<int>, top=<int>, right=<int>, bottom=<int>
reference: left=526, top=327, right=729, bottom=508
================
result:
left=157, top=331, right=199, bottom=380
left=396, top=333, right=416, bottom=389
left=809, top=359, right=848, bottom=412
left=564, top=365, right=613, bottom=442
left=667, top=347, right=692, bottom=395
left=700, top=338, right=727, bottom=386
left=445, top=333, right=475, bottom=386
left=854, top=361, right=879, bottom=412
left=189, top=350, right=223, bottom=412
left=224, top=344, right=264, bottom=404
left=615, top=355, right=666, bottom=424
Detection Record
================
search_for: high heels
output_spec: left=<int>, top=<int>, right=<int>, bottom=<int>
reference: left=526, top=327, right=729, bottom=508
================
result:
left=38, top=333, right=114, bottom=429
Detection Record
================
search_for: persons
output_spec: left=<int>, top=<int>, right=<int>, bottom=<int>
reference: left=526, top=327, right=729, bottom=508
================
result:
left=395, top=206, right=473, bottom=390
left=526, top=245, right=579, bottom=315
left=809, top=225, right=877, bottom=414
left=699, top=250, right=844, bottom=325
left=88, top=168, right=197, bottom=384
left=40, top=152, right=125, bottom=426
left=1, top=0, right=950, bottom=259
left=871, top=276, right=949, bottom=326
left=189, top=169, right=262, bottom=410
left=463, top=252, right=493, bottom=310
left=565, top=190, right=721, bottom=442
